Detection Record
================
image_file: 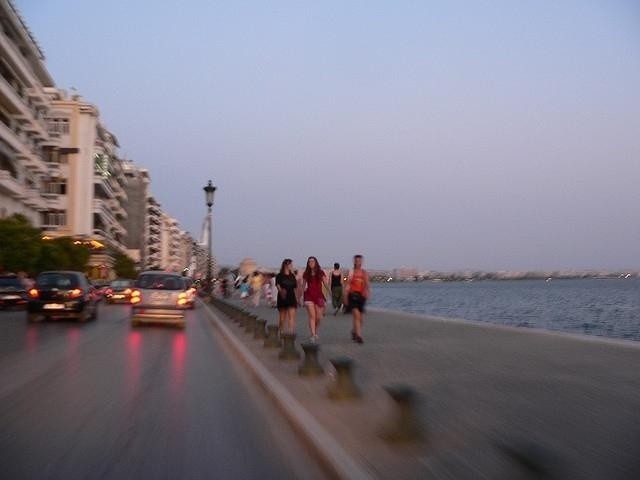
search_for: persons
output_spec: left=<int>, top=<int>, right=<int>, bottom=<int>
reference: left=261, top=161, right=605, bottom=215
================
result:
left=223, top=268, right=330, bottom=308
left=17, top=271, right=36, bottom=290
left=343, top=253, right=372, bottom=342
left=327, top=261, right=345, bottom=316
left=275, top=258, right=297, bottom=336
left=297, top=256, right=334, bottom=338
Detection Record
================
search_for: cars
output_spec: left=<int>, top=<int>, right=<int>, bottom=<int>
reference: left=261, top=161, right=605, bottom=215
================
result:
left=0, top=271, right=197, bottom=329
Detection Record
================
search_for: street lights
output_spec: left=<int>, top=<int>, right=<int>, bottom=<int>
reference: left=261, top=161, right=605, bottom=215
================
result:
left=203, top=180, right=217, bottom=297
